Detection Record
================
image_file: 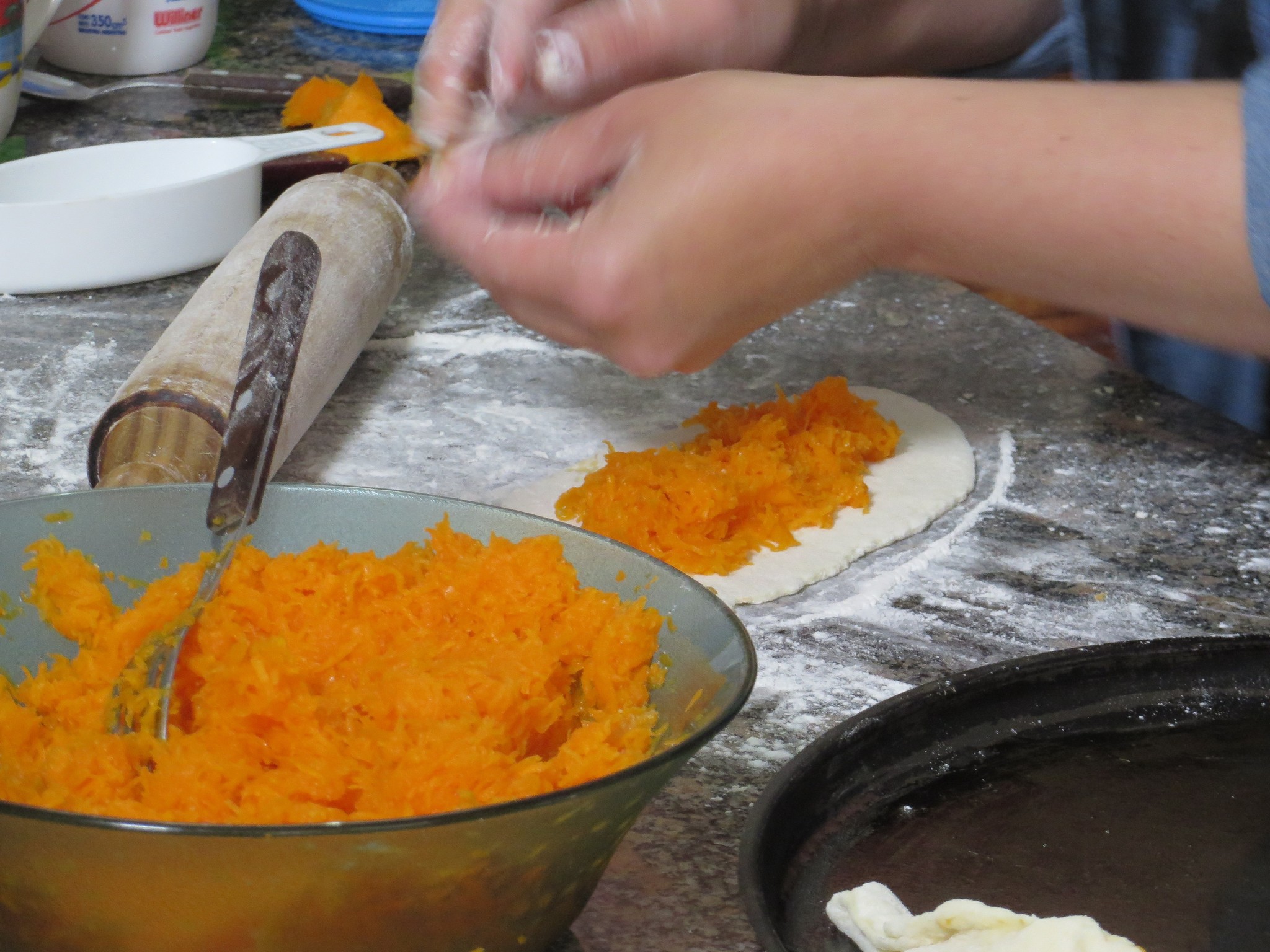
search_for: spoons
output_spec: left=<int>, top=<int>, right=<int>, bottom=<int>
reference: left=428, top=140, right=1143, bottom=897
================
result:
left=18, top=61, right=418, bottom=112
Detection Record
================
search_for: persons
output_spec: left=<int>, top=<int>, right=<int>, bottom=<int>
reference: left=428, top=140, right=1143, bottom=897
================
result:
left=405, top=0, right=1267, bottom=440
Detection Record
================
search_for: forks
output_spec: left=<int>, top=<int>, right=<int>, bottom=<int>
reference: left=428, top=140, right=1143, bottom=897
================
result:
left=99, top=226, right=325, bottom=739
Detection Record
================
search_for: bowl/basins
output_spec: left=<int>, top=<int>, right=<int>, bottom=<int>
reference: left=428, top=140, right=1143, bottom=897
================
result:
left=0, top=485, right=760, bottom=952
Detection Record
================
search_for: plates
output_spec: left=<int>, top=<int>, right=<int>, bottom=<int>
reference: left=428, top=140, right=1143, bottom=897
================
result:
left=745, top=630, right=1269, bottom=951
